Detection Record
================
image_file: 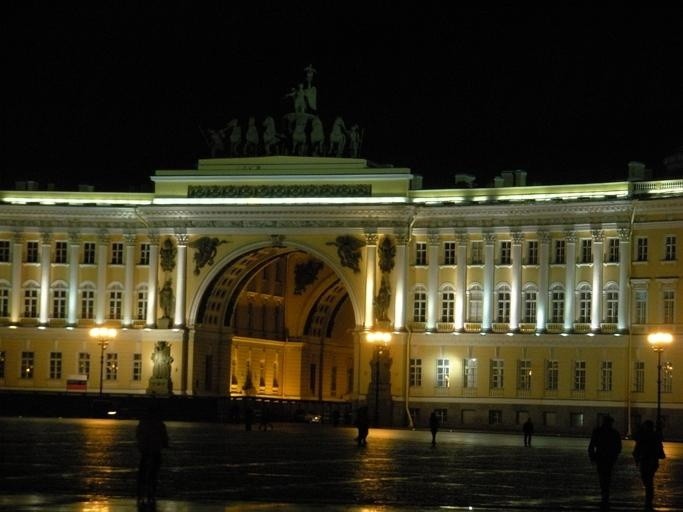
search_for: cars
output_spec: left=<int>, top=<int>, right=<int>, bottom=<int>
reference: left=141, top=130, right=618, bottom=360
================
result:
left=294, top=408, right=321, bottom=425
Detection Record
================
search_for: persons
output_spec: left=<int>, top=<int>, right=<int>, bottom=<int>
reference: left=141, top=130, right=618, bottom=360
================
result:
left=137, top=402, right=170, bottom=510
left=242, top=403, right=308, bottom=433
left=332, top=405, right=369, bottom=444
left=632, top=420, right=665, bottom=511
left=522, top=417, right=533, bottom=445
left=429, top=411, right=438, bottom=444
left=588, top=415, right=622, bottom=509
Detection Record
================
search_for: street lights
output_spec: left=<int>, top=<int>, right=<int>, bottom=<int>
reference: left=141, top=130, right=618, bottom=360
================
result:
left=646, top=329, right=675, bottom=436
left=87, top=326, right=118, bottom=393
left=364, top=329, right=394, bottom=429
